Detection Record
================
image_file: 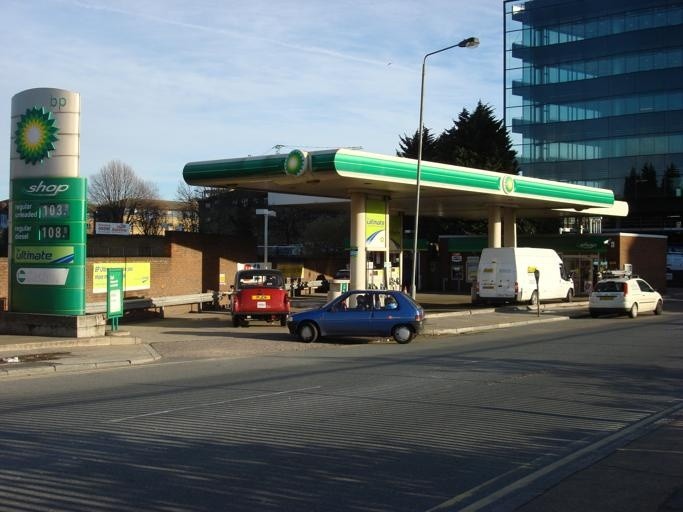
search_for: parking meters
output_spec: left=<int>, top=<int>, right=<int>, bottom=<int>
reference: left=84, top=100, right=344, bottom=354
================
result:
left=535, top=270, right=540, bottom=317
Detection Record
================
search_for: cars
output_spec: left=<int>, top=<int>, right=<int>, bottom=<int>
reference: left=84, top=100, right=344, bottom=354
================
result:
left=589, top=277, right=664, bottom=318
left=287, top=290, right=424, bottom=344
left=229, top=270, right=290, bottom=326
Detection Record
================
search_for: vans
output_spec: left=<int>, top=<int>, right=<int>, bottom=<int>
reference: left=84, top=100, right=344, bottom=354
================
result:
left=477, top=248, right=575, bottom=305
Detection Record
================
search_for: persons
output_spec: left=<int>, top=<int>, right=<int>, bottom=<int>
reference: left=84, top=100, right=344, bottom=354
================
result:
left=363, top=294, right=371, bottom=311
left=384, top=297, right=397, bottom=309
left=355, top=295, right=365, bottom=310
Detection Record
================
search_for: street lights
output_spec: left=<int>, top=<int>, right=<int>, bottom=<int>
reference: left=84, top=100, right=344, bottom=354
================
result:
left=412, top=37, right=480, bottom=301
left=256, top=209, right=276, bottom=270
